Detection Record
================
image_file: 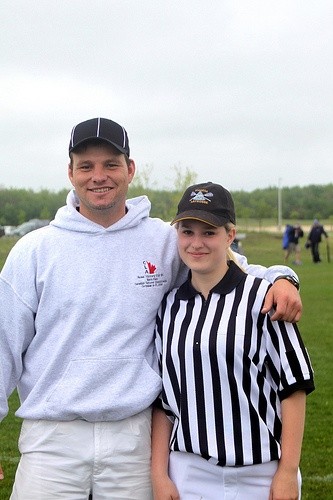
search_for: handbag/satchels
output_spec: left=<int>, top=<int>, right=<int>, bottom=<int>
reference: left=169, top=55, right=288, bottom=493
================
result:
left=305, top=243, right=309, bottom=249
left=282, top=223, right=292, bottom=249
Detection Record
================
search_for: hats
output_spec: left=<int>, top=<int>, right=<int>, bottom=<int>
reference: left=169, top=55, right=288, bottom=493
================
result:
left=69, top=117, right=129, bottom=158
left=170, top=182, right=236, bottom=228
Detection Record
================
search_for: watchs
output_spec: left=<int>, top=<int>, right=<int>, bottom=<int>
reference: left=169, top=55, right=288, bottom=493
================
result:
left=272, top=273, right=301, bottom=291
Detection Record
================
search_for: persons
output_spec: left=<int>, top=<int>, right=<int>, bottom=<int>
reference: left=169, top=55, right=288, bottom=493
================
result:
left=283, top=222, right=304, bottom=265
left=0, top=115, right=304, bottom=500
left=149, top=179, right=318, bottom=500
left=306, top=219, right=330, bottom=265
